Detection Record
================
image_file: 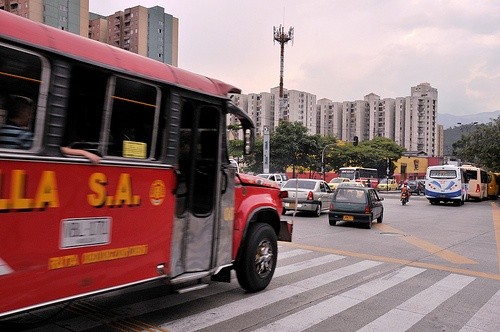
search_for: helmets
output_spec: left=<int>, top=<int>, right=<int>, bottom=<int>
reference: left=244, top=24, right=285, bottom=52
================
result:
left=404, top=182, right=407, bottom=185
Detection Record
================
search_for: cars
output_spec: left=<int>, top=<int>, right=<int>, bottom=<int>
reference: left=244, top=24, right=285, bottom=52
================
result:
left=325, top=177, right=364, bottom=191
left=377, top=179, right=399, bottom=191
left=407, top=179, right=425, bottom=195
left=279, top=178, right=334, bottom=218
left=328, top=186, right=385, bottom=229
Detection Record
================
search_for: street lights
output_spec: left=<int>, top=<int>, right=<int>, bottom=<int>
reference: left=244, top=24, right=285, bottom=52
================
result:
left=457, top=122, right=479, bottom=157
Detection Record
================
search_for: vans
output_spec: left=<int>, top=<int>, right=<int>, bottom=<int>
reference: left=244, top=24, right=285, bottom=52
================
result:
left=257, top=173, right=289, bottom=188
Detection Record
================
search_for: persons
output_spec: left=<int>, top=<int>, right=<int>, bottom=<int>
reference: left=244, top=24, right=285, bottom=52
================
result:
left=61, top=146, right=100, bottom=164
left=400, top=181, right=410, bottom=202
left=0, top=96, right=34, bottom=164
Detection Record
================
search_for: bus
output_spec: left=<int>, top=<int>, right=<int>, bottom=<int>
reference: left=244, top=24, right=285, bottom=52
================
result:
left=424, top=164, right=500, bottom=206
left=338, top=166, right=379, bottom=190
left=0, top=8, right=294, bottom=321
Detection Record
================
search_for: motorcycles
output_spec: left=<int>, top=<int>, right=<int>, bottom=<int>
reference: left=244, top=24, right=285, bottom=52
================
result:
left=400, top=189, right=411, bottom=206
